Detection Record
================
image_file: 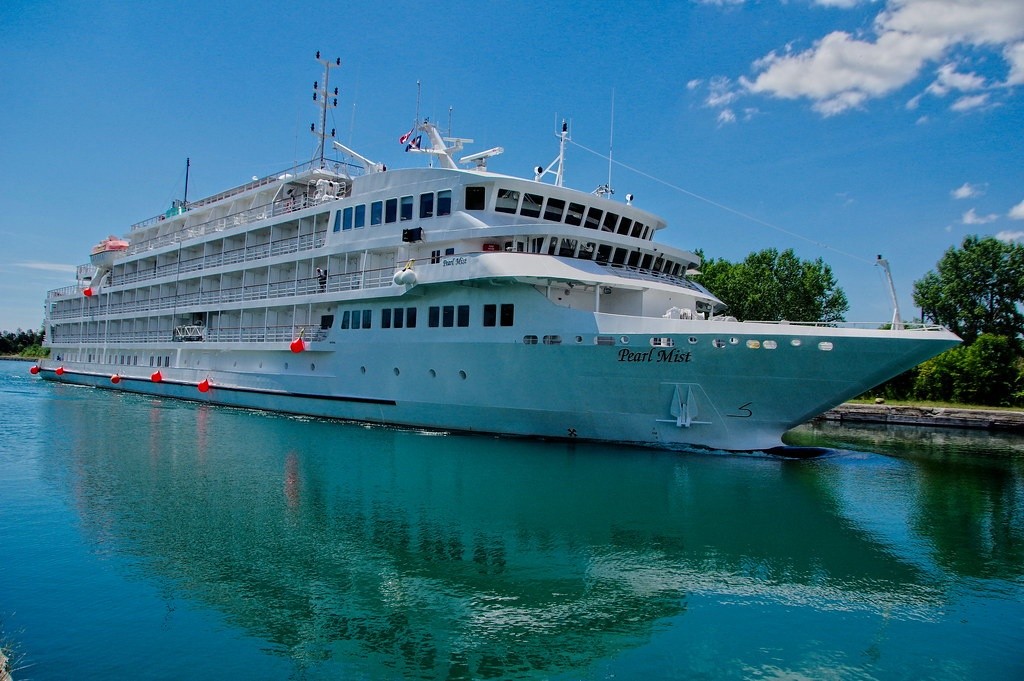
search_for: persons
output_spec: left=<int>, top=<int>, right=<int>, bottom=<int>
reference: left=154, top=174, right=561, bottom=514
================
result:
left=316, top=267, right=326, bottom=292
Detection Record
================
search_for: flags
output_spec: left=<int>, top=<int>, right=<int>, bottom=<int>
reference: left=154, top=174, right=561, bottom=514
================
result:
left=405, top=135, right=421, bottom=152
left=400, top=128, right=414, bottom=144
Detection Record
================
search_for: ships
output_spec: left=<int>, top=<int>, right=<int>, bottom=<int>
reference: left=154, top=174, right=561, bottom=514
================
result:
left=31, top=48, right=965, bottom=453
